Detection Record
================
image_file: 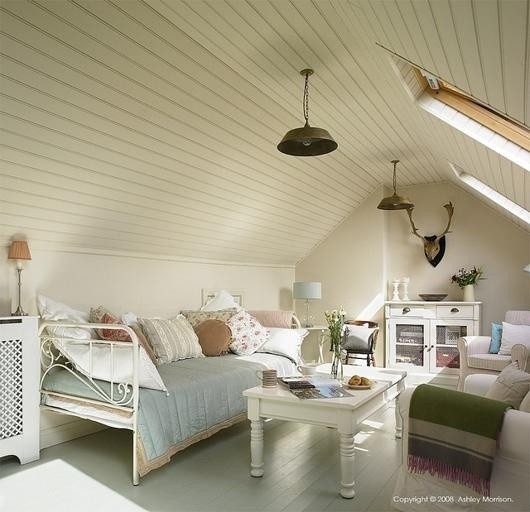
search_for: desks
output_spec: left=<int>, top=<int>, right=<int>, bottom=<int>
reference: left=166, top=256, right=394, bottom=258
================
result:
left=305, top=325, right=328, bottom=363
left=1, top=315, right=41, bottom=466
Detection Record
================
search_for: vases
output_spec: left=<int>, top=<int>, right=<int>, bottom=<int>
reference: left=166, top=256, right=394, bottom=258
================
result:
left=330, top=341, right=346, bottom=377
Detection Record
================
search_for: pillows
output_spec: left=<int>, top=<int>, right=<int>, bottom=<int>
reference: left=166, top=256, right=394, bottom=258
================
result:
left=489, top=321, right=503, bottom=353
left=37, top=290, right=310, bottom=391
left=485, top=360, right=530, bottom=409
left=499, top=321, right=530, bottom=356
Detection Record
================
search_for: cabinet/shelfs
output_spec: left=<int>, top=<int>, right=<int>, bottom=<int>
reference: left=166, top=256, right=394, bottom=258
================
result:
left=385, top=300, right=484, bottom=375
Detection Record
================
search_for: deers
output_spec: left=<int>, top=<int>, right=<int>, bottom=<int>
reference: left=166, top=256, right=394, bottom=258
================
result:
left=407, top=201, right=454, bottom=261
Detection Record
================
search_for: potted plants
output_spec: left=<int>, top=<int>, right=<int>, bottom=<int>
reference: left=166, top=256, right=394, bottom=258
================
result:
left=451, top=265, right=488, bottom=301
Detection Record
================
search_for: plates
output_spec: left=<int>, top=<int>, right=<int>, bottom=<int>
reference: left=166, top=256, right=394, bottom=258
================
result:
left=341, top=381, right=378, bottom=390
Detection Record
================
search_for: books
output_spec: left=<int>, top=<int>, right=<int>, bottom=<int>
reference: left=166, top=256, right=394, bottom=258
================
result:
left=280, top=375, right=353, bottom=401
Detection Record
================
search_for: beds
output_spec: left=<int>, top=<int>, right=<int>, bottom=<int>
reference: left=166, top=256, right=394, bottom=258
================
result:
left=38, top=310, right=302, bottom=486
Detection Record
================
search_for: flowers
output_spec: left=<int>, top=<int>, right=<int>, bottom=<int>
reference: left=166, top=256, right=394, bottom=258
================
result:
left=324, top=304, right=347, bottom=377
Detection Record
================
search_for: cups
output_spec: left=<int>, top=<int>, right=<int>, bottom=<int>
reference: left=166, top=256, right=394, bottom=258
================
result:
left=255, top=369, right=278, bottom=387
left=297, top=365, right=316, bottom=382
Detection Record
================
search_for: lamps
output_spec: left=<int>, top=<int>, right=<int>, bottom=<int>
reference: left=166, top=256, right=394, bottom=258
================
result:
left=8, top=240, right=32, bottom=315
left=293, top=281, right=322, bottom=328
left=377, top=160, right=414, bottom=210
left=277, top=69, right=338, bottom=157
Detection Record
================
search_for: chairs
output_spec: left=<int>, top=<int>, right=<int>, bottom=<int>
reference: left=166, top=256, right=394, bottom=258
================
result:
left=400, top=374, right=530, bottom=512
left=457, top=310, right=530, bottom=393
left=345, top=320, right=380, bottom=366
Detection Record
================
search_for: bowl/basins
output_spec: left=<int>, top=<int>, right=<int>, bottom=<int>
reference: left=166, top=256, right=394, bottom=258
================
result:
left=418, top=293, right=448, bottom=301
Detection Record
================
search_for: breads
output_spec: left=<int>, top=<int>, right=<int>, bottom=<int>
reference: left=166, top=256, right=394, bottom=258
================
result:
left=348, top=375, right=361, bottom=385
left=361, top=377, right=370, bottom=385
